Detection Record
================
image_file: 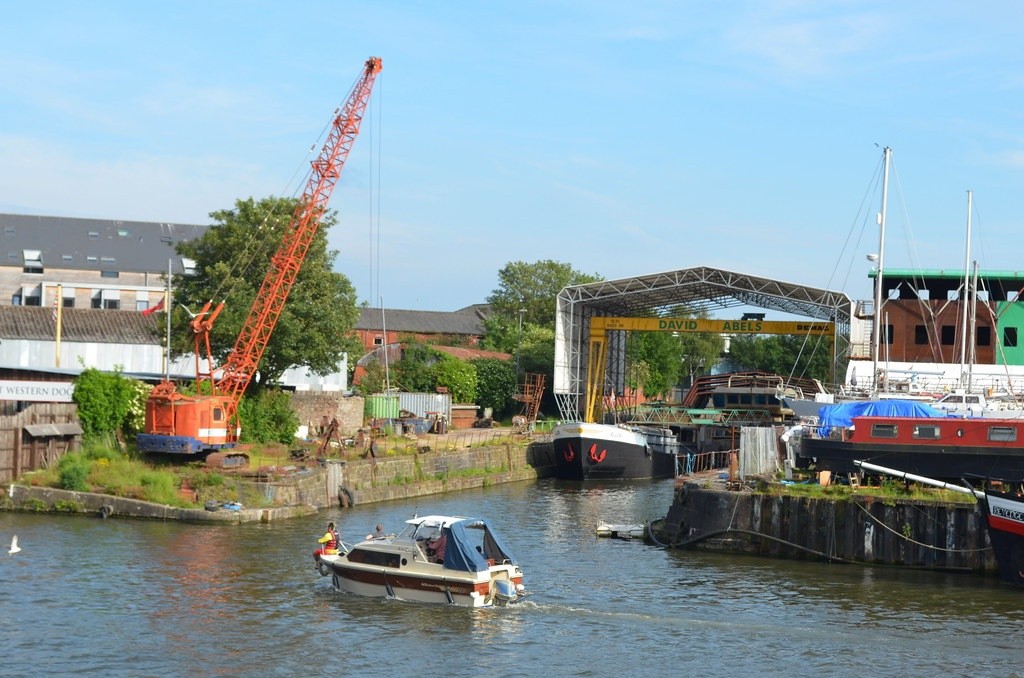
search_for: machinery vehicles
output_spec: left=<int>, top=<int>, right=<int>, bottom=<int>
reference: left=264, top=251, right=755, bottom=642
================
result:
left=137, top=54, right=384, bottom=473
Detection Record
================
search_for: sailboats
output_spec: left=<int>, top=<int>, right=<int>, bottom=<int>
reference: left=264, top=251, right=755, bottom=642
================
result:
left=774, top=143, right=1023, bottom=487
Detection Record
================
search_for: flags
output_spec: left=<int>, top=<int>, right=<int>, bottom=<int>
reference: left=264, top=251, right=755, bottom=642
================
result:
left=142, top=297, right=164, bottom=317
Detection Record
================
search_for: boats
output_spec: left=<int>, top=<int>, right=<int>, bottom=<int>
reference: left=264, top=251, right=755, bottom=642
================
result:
left=552, top=421, right=681, bottom=488
left=315, top=514, right=527, bottom=610
left=962, top=456, right=1024, bottom=587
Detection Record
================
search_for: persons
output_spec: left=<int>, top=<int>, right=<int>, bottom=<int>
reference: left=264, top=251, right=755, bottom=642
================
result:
left=313, top=522, right=338, bottom=570
left=375, top=523, right=386, bottom=537
left=427, top=529, right=448, bottom=564
